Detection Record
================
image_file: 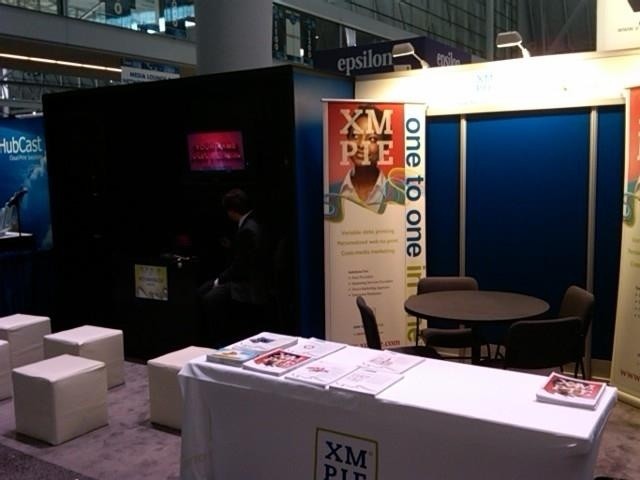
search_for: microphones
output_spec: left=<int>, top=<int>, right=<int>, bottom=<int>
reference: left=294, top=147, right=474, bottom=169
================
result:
left=7, top=188, right=28, bottom=208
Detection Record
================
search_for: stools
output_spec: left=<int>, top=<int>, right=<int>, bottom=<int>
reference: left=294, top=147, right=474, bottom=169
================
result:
left=146, top=345, right=219, bottom=430
left=43, top=323, right=125, bottom=389
left=11, top=353, right=109, bottom=445
left=0, top=313, right=52, bottom=367
left=0, top=339, right=14, bottom=402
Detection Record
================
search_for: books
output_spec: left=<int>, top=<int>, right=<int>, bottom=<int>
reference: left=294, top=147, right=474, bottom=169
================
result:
left=535, top=371, right=607, bottom=408
left=364, top=348, right=424, bottom=375
left=330, top=365, right=404, bottom=398
left=285, top=346, right=383, bottom=390
left=207, top=331, right=347, bottom=377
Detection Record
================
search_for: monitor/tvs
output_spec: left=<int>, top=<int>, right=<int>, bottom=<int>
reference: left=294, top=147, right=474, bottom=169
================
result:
left=134, top=264, right=168, bottom=301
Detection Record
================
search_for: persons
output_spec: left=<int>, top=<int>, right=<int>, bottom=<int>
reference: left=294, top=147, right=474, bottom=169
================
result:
left=327, top=105, right=403, bottom=209
left=212, top=184, right=260, bottom=290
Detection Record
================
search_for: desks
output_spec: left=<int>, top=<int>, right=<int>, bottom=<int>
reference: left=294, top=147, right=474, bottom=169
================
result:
left=177, top=330, right=619, bottom=480
left=403, top=290, right=550, bottom=366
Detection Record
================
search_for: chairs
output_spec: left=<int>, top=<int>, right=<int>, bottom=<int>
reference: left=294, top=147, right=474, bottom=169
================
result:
left=485, top=318, right=585, bottom=380
left=415, top=276, right=492, bottom=359
left=505, top=286, right=595, bottom=380
left=356, top=294, right=442, bottom=358
